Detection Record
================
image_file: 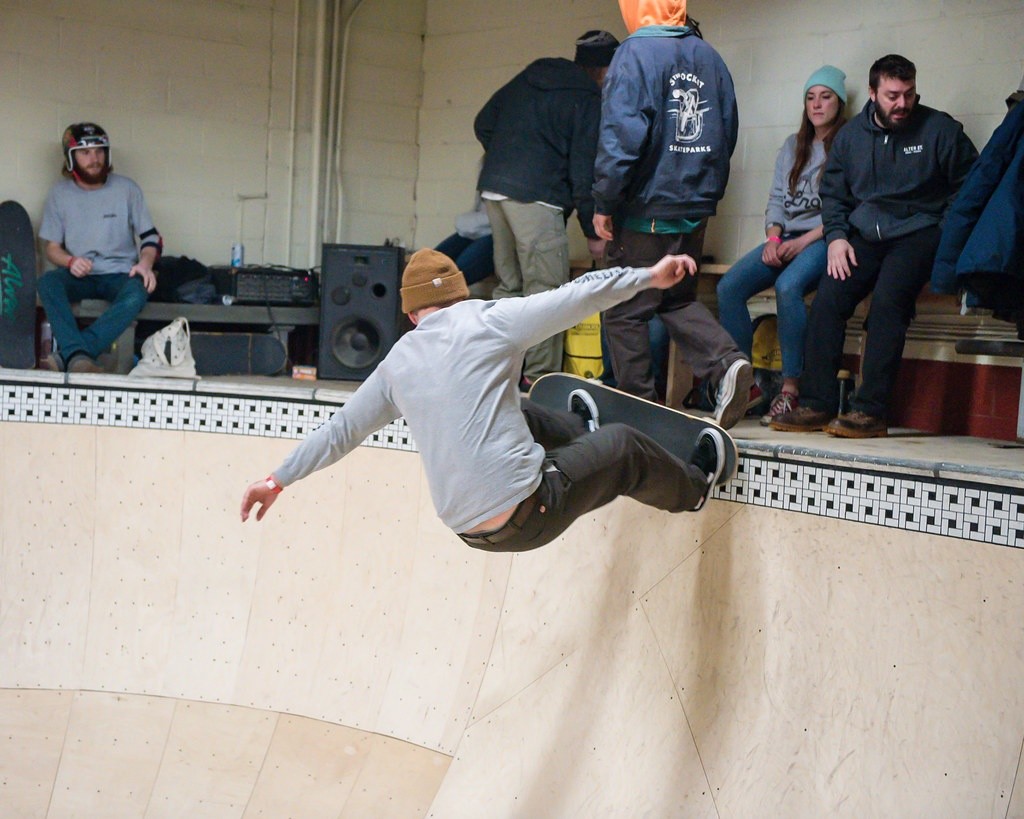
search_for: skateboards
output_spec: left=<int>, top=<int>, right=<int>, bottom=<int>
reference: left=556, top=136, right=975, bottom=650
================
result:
left=0, top=199, right=40, bottom=370
left=525, top=370, right=741, bottom=487
left=133, top=332, right=288, bottom=378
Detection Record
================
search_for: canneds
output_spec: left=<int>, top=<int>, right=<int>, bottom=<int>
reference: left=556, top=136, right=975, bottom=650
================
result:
left=231, top=242, right=243, bottom=267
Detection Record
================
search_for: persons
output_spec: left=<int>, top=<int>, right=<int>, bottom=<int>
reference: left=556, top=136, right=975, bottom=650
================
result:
left=240, top=249, right=727, bottom=552
left=716, top=66, right=848, bottom=423
left=435, top=152, right=496, bottom=287
left=473, top=29, right=620, bottom=394
left=35, top=123, right=162, bottom=372
left=591, top=0, right=754, bottom=432
left=768, top=55, right=981, bottom=437
left=597, top=308, right=671, bottom=407
left=930, top=73, right=1024, bottom=341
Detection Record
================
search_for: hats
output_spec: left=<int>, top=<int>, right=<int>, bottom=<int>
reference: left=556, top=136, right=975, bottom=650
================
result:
left=399, top=247, right=470, bottom=314
left=802, top=64, right=847, bottom=107
left=572, top=31, right=621, bottom=67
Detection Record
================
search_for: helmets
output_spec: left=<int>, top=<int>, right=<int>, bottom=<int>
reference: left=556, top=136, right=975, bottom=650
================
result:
left=61, top=123, right=112, bottom=172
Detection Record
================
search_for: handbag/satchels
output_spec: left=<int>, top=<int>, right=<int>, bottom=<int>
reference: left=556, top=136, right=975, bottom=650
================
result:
left=145, top=254, right=226, bottom=305
left=129, top=315, right=199, bottom=376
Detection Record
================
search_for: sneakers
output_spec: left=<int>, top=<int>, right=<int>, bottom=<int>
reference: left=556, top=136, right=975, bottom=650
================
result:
left=568, top=389, right=600, bottom=434
left=769, top=403, right=836, bottom=431
left=744, top=384, right=765, bottom=407
left=761, top=392, right=799, bottom=425
left=828, top=410, right=888, bottom=437
left=686, top=429, right=726, bottom=511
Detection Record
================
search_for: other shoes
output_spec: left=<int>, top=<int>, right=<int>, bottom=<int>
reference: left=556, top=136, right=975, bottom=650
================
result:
left=65, top=353, right=99, bottom=371
left=47, top=350, right=64, bottom=371
left=715, top=359, right=752, bottom=431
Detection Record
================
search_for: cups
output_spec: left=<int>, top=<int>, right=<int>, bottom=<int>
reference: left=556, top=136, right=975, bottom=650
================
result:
left=832, top=378, right=855, bottom=417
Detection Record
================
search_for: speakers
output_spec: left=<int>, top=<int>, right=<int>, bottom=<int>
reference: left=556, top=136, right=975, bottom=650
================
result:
left=316, top=244, right=406, bottom=380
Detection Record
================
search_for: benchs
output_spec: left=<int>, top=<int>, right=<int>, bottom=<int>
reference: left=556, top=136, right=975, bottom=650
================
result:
left=667, top=264, right=888, bottom=427
left=33, top=293, right=320, bottom=380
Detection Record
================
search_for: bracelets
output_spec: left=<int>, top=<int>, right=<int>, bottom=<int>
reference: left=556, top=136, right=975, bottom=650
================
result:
left=66, top=256, right=73, bottom=268
left=765, top=236, right=783, bottom=244
left=265, top=475, right=283, bottom=494
